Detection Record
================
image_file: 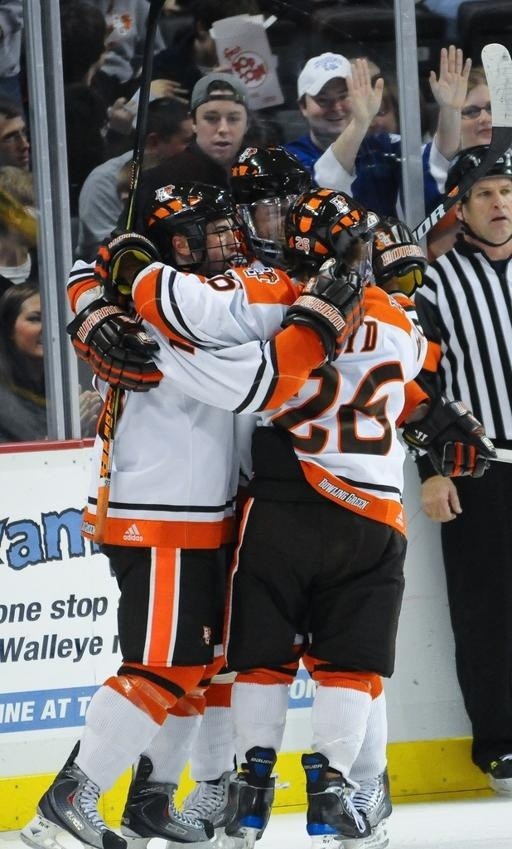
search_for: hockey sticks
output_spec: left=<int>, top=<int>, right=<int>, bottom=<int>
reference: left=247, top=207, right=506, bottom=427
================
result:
left=408, top=43, right=512, bottom=240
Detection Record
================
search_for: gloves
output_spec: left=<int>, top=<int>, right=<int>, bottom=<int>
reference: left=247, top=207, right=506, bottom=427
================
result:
left=282, top=233, right=366, bottom=368
left=403, top=388, right=496, bottom=479
left=66, top=293, right=164, bottom=392
left=93, top=227, right=160, bottom=297
left=372, top=215, right=428, bottom=293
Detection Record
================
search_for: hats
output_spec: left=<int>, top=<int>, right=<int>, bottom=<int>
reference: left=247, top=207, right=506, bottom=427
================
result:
left=190, top=73, right=251, bottom=111
left=296, top=52, right=352, bottom=102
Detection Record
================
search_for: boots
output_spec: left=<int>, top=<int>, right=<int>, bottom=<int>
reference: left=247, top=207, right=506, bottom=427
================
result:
left=225, top=746, right=277, bottom=839
left=36, top=741, right=127, bottom=849
left=181, top=755, right=238, bottom=828
left=301, top=752, right=371, bottom=837
left=350, top=766, right=393, bottom=827
left=120, top=751, right=214, bottom=843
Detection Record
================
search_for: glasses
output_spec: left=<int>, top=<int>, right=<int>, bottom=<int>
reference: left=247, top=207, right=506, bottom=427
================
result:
left=460, top=101, right=492, bottom=120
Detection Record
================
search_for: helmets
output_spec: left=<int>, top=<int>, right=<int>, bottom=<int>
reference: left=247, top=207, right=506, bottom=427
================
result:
left=284, top=187, right=381, bottom=276
left=137, top=181, right=245, bottom=272
left=445, top=146, right=512, bottom=196
left=230, top=143, right=312, bottom=201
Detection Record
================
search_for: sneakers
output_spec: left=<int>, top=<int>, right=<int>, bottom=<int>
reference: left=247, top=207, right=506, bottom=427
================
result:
left=487, top=753, right=512, bottom=781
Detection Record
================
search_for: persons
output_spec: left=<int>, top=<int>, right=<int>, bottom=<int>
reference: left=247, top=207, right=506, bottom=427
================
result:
left=34, top=178, right=365, bottom=849
left=65, top=143, right=495, bottom=838
left=95, top=187, right=428, bottom=840
left=402, top=141, right=512, bottom=780
